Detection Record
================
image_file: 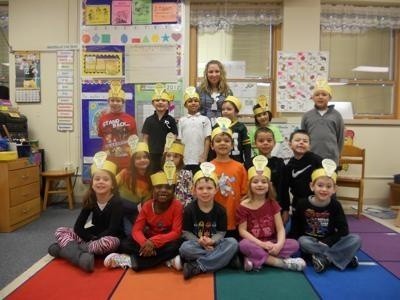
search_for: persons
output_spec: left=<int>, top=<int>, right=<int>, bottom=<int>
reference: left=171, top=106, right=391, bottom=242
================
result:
left=48, top=160, right=126, bottom=272
left=235, top=165, right=307, bottom=273
left=297, top=168, right=364, bottom=273
left=20, top=57, right=39, bottom=88
left=175, top=92, right=213, bottom=174
left=115, top=142, right=154, bottom=236
left=96, top=88, right=138, bottom=156
left=103, top=171, right=186, bottom=272
left=140, top=90, right=179, bottom=174
left=206, top=95, right=252, bottom=170
left=203, top=123, right=250, bottom=243
left=248, top=101, right=284, bottom=159
left=300, top=84, right=344, bottom=177
left=241, top=127, right=290, bottom=228
left=196, top=60, right=234, bottom=120
left=285, top=129, right=323, bottom=239
left=159, top=140, right=197, bottom=212
left=178, top=169, right=240, bottom=280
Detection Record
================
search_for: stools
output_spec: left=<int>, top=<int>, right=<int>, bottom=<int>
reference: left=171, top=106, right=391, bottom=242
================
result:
left=40, top=170, right=75, bottom=211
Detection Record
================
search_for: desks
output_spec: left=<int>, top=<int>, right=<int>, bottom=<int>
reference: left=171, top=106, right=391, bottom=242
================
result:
left=388, top=181, right=400, bottom=208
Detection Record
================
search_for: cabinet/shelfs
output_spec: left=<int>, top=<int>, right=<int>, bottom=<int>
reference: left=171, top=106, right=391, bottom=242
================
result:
left=1, top=154, right=43, bottom=233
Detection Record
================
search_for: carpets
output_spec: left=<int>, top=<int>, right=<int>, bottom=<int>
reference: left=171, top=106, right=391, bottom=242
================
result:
left=1, top=199, right=97, bottom=292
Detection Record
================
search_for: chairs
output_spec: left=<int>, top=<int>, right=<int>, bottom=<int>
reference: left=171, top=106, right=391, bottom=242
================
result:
left=333, top=144, right=365, bottom=216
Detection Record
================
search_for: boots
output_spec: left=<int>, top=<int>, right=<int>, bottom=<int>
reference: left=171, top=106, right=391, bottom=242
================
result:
left=60, top=240, right=94, bottom=271
left=48, top=242, right=89, bottom=259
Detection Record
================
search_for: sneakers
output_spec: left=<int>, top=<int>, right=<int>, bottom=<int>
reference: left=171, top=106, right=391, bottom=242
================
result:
left=244, top=257, right=253, bottom=271
left=311, top=254, right=329, bottom=272
left=103, top=253, right=132, bottom=270
left=348, top=256, right=358, bottom=268
left=283, top=257, right=306, bottom=272
left=166, top=255, right=183, bottom=271
left=229, top=255, right=240, bottom=269
left=184, top=262, right=202, bottom=279
